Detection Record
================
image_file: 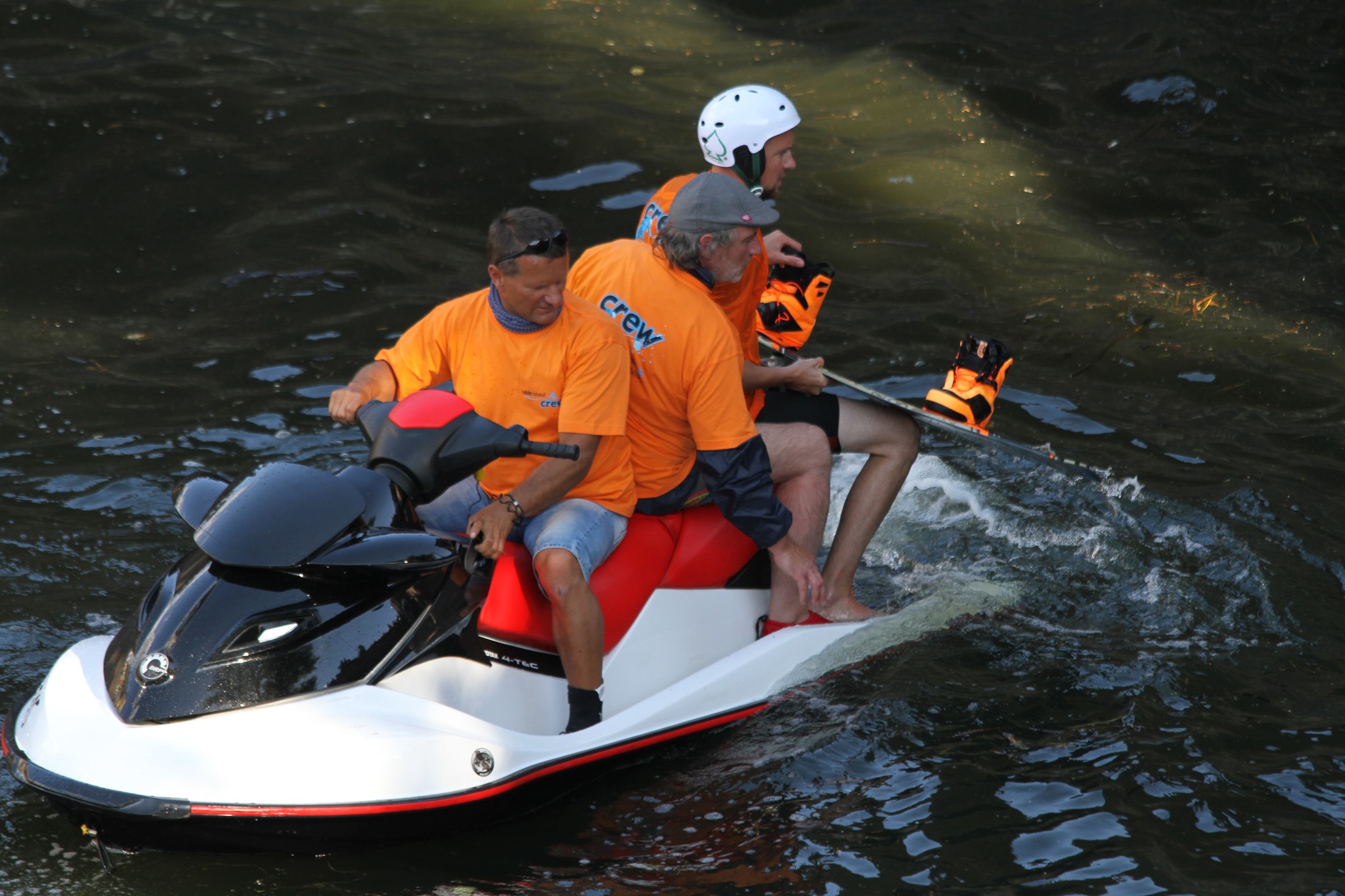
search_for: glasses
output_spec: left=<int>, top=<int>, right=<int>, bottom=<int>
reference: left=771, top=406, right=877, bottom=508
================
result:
left=494, top=228, right=570, bottom=266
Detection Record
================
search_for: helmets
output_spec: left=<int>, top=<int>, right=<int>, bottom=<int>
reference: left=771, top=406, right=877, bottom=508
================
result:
left=697, top=84, right=800, bottom=182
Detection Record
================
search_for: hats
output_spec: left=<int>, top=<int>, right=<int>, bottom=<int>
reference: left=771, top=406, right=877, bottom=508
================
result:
left=668, top=172, right=780, bottom=233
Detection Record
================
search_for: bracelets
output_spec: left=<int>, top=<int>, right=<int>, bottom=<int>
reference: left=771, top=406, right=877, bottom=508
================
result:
left=491, top=493, right=526, bottom=527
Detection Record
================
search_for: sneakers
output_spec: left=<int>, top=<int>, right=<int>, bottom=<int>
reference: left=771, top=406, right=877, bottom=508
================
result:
left=756, top=246, right=836, bottom=352
left=922, top=335, right=1013, bottom=429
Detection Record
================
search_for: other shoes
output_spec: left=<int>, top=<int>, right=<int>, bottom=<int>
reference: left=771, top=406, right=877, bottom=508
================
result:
left=755, top=609, right=834, bottom=643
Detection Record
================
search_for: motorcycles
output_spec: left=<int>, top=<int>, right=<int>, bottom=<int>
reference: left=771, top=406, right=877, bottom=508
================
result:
left=0, top=387, right=893, bottom=822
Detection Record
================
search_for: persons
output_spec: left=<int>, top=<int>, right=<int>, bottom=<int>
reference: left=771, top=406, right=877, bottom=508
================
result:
left=634, top=83, right=923, bottom=625
left=327, top=205, right=638, bottom=738
left=565, top=170, right=831, bottom=641
